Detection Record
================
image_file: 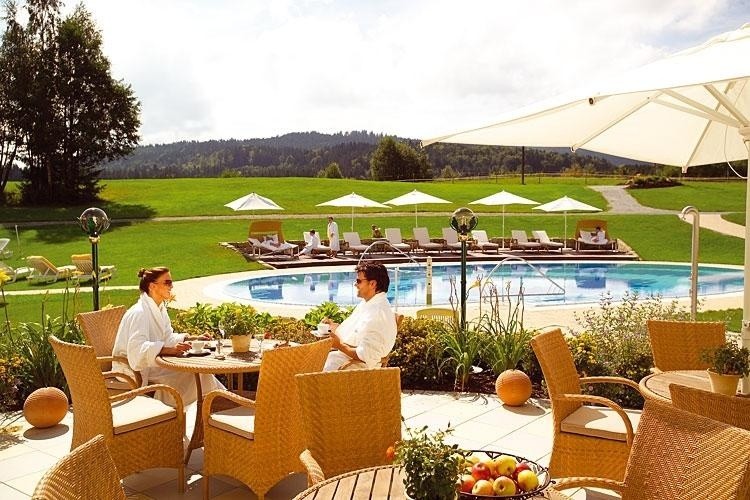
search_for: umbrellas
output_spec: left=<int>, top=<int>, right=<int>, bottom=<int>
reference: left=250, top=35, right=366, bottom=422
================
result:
left=468, top=190, right=542, bottom=247
left=419, top=19, right=750, bottom=350
left=314, top=192, right=392, bottom=231
left=223, top=192, right=286, bottom=238
left=531, top=195, right=604, bottom=248
left=382, top=189, right=453, bottom=228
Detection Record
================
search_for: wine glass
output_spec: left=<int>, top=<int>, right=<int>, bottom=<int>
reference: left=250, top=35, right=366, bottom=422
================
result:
left=253, top=331, right=265, bottom=356
left=217, top=319, right=227, bottom=344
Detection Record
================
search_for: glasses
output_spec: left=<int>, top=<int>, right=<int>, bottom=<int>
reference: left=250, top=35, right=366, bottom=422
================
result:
left=151, top=281, right=174, bottom=286
left=356, top=277, right=369, bottom=284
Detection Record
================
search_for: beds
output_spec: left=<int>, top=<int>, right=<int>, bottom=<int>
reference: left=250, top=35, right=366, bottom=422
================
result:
left=573, top=219, right=619, bottom=255
left=247, top=218, right=301, bottom=263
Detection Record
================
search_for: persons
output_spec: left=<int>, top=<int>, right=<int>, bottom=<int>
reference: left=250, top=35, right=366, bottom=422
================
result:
left=598, top=227, right=605, bottom=241
left=322, top=262, right=398, bottom=371
left=111, top=266, right=228, bottom=408
left=590, top=226, right=600, bottom=243
left=327, top=216, right=340, bottom=258
left=294, top=230, right=321, bottom=257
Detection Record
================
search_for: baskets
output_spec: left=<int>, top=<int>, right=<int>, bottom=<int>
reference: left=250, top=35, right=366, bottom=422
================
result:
left=454, top=449, right=550, bottom=499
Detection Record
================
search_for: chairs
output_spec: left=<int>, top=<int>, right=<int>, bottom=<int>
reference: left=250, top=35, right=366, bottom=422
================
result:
left=530, top=319, right=750, bottom=498
left=0, top=236, right=116, bottom=286
left=302, top=226, right=566, bottom=260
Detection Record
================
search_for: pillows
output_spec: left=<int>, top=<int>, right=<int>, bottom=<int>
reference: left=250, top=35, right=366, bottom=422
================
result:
left=249, top=237, right=262, bottom=245
left=262, top=234, right=279, bottom=244
left=580, top=229, right=605, bottom=240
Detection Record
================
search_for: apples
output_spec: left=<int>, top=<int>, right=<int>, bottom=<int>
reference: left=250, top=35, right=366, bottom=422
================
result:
left=460, top=455, right=539, bottom=496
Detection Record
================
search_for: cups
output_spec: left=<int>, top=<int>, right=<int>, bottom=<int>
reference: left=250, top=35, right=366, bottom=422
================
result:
left=317, top=324, right=329, bottom=332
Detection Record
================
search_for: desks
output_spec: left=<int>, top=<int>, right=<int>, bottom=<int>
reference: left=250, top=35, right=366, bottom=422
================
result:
left=289, top=463, right=579, bottom=500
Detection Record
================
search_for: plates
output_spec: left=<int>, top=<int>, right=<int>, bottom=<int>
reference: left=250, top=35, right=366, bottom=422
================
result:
left=310, top=330, right=329, bottom=338
left=180, top=340, right=223, bottom=356
